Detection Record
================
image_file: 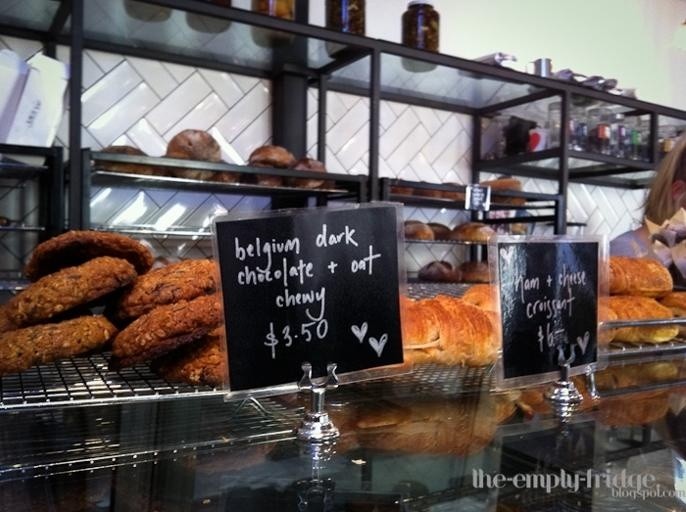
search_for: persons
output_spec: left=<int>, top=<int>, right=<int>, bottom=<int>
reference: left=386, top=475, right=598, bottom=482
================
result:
left=608, top=135, right=685, bottom=259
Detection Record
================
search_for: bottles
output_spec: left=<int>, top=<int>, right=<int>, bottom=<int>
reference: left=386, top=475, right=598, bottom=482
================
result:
left=544, top=102, right=651, bottom=161
left=123, top=0, right=364, bottom=58
left=401, top=2, right=441, bottom=72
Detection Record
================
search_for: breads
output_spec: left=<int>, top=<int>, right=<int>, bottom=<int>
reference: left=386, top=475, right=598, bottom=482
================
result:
left=325, top=360, right=686, bottom=455
left=96, top=129, right=686, bottom=372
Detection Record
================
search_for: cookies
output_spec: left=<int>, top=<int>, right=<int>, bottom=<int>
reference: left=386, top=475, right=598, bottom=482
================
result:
left=0, top=231, right=228, bottom=384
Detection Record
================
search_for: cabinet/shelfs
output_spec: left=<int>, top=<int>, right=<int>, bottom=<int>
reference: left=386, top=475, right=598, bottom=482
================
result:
left=474, top=69, right=686, bottom=195
left=0, top=0, right=571, bottom=297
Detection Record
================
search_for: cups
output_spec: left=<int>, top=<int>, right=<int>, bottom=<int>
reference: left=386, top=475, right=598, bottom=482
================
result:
left=534, top=58, right=552, bottom=77
